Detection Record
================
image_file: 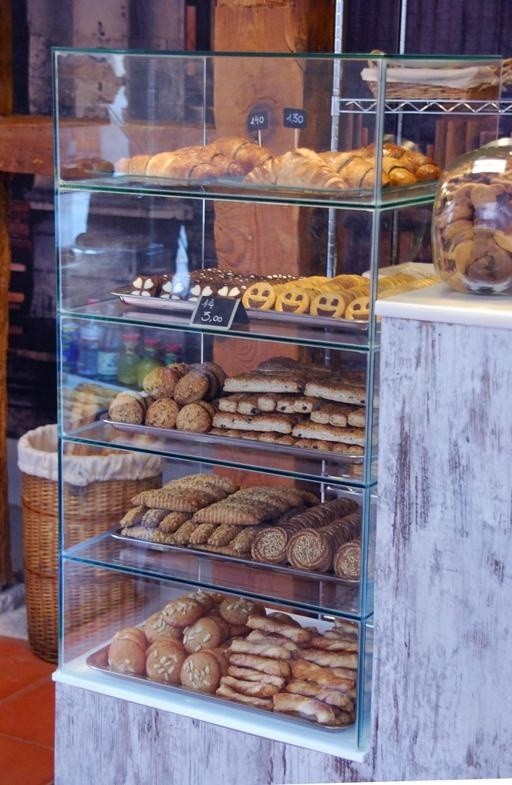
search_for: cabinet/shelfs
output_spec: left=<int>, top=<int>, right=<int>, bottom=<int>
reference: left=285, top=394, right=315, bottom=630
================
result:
left=48, top=45, right=512, bottom=782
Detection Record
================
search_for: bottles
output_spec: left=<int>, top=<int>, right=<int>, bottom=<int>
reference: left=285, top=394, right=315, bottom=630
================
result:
left=60, top=296, right=182, bottom=393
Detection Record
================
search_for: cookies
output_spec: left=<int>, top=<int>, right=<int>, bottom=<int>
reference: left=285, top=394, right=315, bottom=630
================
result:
left=129, top=258, right=298, bottom=303
left=242, top=275, right=438, bottom=321
left=433, top=163, right=511, bottom=293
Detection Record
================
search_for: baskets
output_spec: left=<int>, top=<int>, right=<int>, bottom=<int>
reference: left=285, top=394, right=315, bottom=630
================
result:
left=361, top=50, right=511, bottom=101
left=18, top=419, right=166, bottom=665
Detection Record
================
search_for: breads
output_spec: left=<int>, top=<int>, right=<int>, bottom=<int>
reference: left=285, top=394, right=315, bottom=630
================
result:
left=107, top=356, right=379, bottom=459
left=121, top=475, right=375, bottom=580
left=112, top=137, right=441, bottom=190
left=107, top=591, right=371, bottom=731
